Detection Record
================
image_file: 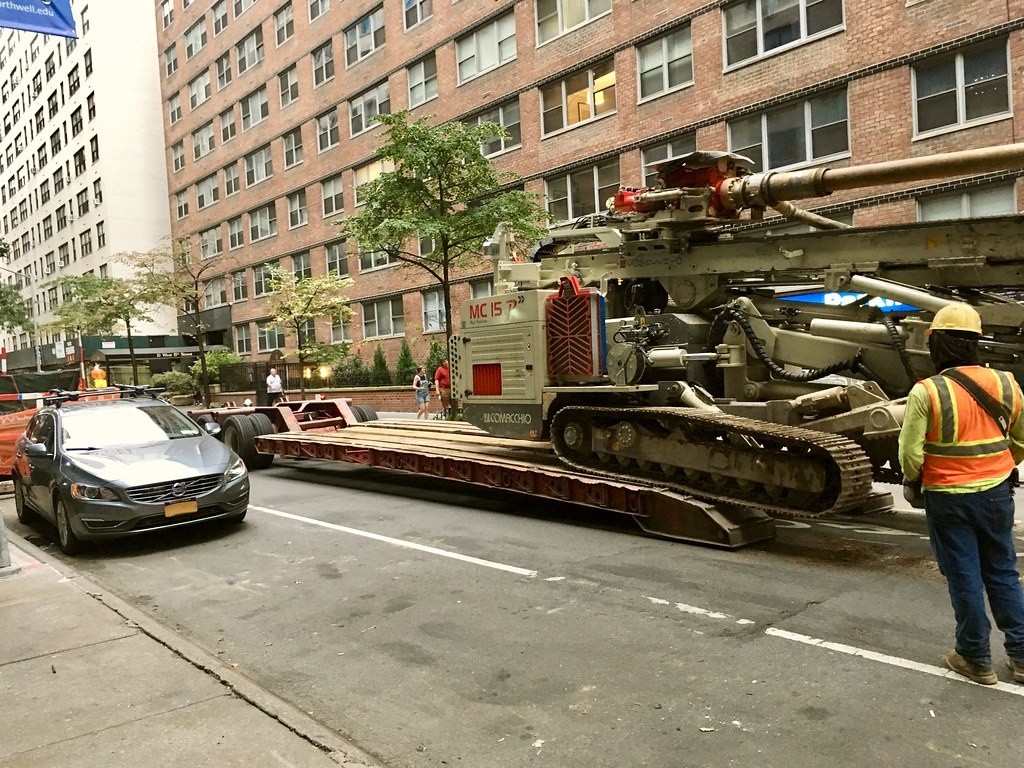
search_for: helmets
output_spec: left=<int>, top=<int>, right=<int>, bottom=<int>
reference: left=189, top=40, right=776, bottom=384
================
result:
left=924, top=302, right=984, bottom=335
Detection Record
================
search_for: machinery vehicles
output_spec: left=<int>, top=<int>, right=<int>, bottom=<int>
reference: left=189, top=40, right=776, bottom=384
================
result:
left=447, top=143, right=1024, bottom=520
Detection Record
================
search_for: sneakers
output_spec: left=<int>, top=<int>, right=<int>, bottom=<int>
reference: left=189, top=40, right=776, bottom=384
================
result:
left=1006, top=656, right=1024, bottom=683
left=946, top=653, right=998, bottom=684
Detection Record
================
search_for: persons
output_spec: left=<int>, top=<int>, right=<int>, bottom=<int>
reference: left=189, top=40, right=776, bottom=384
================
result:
left=413, top=365, right=430, bottom=420
left=434, top=357, right=450, bottom=408
left=266, top=368, right=283, bottom=407
left=897, top=302, right=1024, bottom=685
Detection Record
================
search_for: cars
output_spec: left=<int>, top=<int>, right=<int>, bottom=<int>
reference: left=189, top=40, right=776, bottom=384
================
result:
left=11, top=383, right=250, bottom=559
